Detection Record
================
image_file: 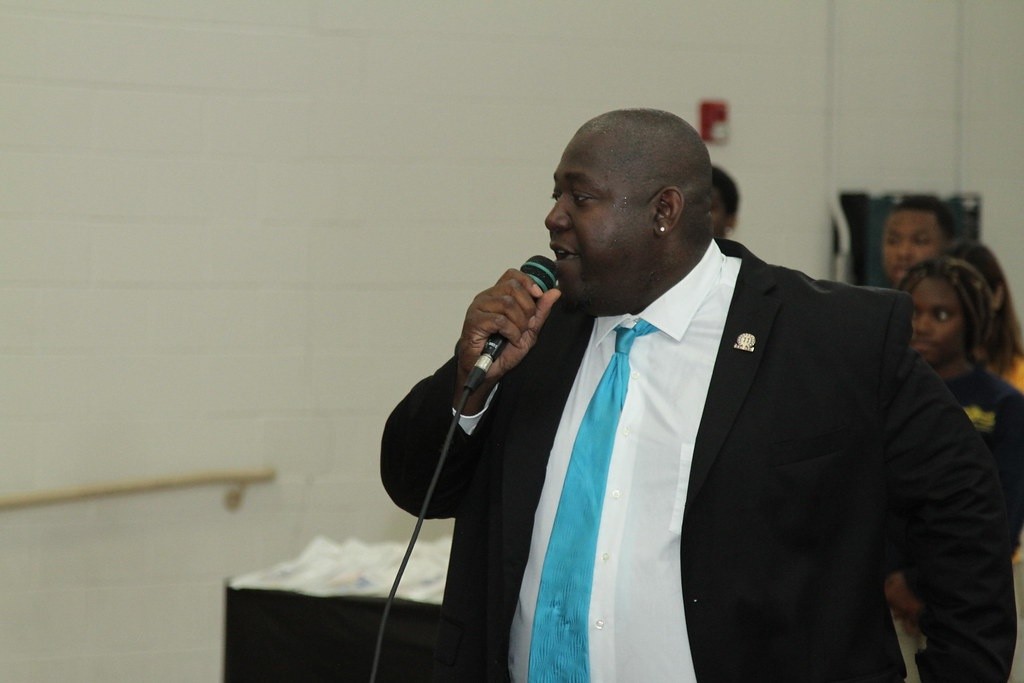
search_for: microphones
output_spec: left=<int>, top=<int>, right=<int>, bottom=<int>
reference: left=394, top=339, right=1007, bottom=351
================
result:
left=463, top=255, right=559, bottom=395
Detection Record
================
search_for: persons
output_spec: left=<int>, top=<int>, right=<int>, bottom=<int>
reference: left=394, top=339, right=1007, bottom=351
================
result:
left=894, top=240, right=1024, bottom=683
left=382, top=109, right=1017, bottom=683
left=883, top=197, right=955, bottom=288
left=711, top=166, right=739, bottom=239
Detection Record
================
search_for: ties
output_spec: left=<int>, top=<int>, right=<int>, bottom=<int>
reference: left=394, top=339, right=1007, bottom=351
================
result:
left=525, top=317, right=662, bottom=683
